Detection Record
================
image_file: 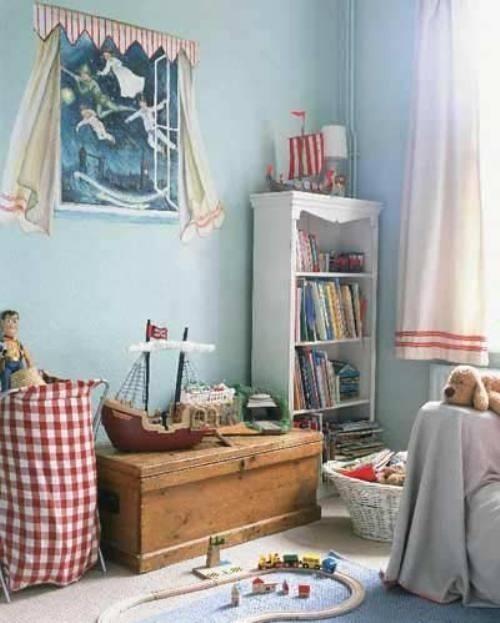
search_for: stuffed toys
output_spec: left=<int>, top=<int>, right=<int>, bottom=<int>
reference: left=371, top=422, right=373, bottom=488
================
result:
left=440, top=362, right=500, bottom=414
left=373, top=459, right=407, bottom=485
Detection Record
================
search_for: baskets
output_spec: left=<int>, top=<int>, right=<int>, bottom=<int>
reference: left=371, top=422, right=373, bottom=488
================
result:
left=323, top=459, right=403, bottom=543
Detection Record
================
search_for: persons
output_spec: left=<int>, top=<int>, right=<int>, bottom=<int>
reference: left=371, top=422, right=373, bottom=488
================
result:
left=75, top=101, right=117, bottom=146
left=93, top=46, right=148, bottom=107
left=122, top=96, right=178, bottom=154
left=71, top=63, right=136, bottom=119
left=0, top=309, right=33, bottom=391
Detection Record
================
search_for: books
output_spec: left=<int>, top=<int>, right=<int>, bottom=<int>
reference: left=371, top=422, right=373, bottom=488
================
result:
left=293, top=224, right=384, bottom=487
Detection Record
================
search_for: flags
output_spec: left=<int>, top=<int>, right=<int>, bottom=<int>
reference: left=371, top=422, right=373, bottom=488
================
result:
left=291, top=110, right=306, bottom=120
left=144, top=318, right=169, bottom=340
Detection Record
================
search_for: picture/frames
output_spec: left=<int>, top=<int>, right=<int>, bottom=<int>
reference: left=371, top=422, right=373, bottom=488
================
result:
left=53, top=30, right=186, bottom=220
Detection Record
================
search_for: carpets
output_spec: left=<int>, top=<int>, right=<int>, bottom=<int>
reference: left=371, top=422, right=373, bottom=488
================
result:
left=136, top=550, right=500, bottom=623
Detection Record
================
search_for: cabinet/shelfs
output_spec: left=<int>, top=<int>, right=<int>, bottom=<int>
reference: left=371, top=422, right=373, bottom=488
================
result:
left=248, top=189, right=384, bottom=503
left=96, top=430, right=323, bottom=572
